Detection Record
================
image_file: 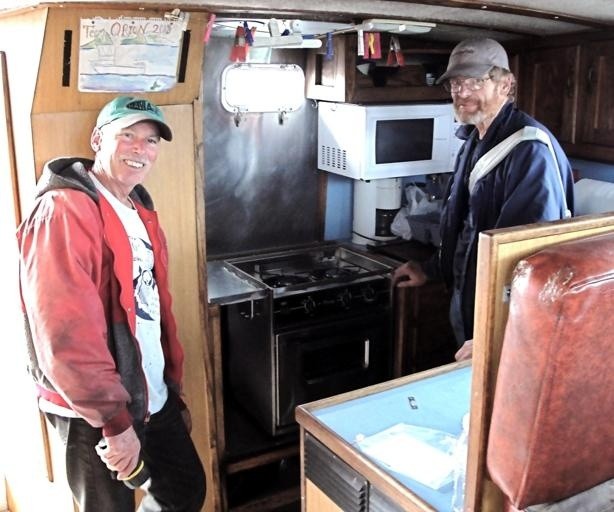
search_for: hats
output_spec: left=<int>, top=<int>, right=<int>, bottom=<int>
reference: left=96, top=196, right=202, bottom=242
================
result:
left=95, top=94, right=172, bottom=143
left=436, top=38, right=511, bottom=84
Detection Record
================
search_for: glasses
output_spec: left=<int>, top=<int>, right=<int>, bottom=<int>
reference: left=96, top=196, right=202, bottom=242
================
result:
left=443, top=75, right=496, bottom=91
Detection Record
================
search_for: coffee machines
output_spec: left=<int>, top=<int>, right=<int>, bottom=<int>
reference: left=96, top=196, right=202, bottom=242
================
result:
left=348, top=177, right=406, bottom=243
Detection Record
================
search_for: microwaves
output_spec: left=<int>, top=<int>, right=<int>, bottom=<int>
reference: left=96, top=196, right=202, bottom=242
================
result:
left=315, top=101, right=466, bottom=180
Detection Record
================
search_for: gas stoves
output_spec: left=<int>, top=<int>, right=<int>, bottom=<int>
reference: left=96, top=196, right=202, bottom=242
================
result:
left=227, top=246, right=395, bottom=324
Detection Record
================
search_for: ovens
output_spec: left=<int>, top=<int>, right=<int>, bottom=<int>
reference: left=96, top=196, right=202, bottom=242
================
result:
left=221, top=312, right=392, bottom=447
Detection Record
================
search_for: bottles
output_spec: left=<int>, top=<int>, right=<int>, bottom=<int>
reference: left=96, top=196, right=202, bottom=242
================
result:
left=413, top=175, right=445, bottom=207
left=98, top=437, right=154, bottom=495
left=425, top=72, right=435, bottom=87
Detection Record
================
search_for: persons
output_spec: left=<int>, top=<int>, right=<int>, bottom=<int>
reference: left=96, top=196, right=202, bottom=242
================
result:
left=394, top=38, right=575, bottom=363
left=15, top=94, right=207, bottom=511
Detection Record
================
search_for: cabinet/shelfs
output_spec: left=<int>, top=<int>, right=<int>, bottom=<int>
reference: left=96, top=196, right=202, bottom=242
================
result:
left=304, top=33, right=451, bottom=104
left=506, top=27, right=614, bottom=164
left=391, top=277, right=460, bottom=379
